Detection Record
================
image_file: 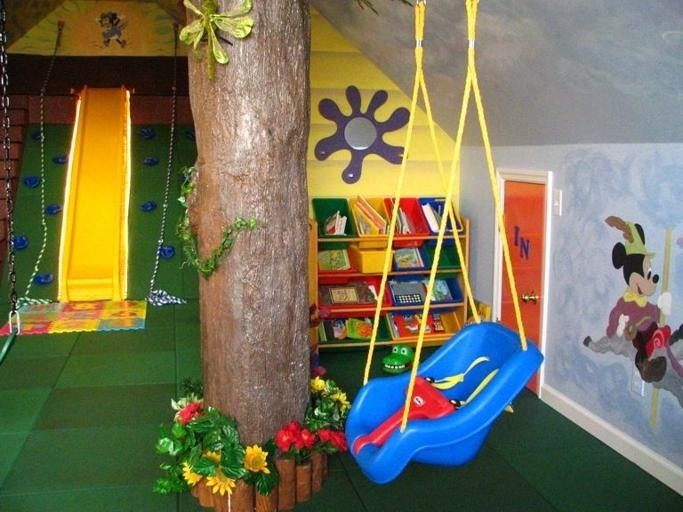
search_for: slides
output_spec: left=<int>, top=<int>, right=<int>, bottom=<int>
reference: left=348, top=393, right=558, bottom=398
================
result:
left=58, top=83, right=132, bottom=301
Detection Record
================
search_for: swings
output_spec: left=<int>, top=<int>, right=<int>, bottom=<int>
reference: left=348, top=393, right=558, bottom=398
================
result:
left=345, top=3, right=543, bottom=483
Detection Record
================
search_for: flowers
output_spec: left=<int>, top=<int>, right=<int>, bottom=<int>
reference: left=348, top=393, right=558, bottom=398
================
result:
left=150, top=366, right=353, bottom=496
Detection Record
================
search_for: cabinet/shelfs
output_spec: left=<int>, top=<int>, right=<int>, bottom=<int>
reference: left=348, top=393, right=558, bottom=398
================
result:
left=306, top=215, right=470, bottom=365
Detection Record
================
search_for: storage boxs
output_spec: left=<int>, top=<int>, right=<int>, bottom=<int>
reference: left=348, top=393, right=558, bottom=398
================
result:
left=313, top=197, right=464, bottom=353
